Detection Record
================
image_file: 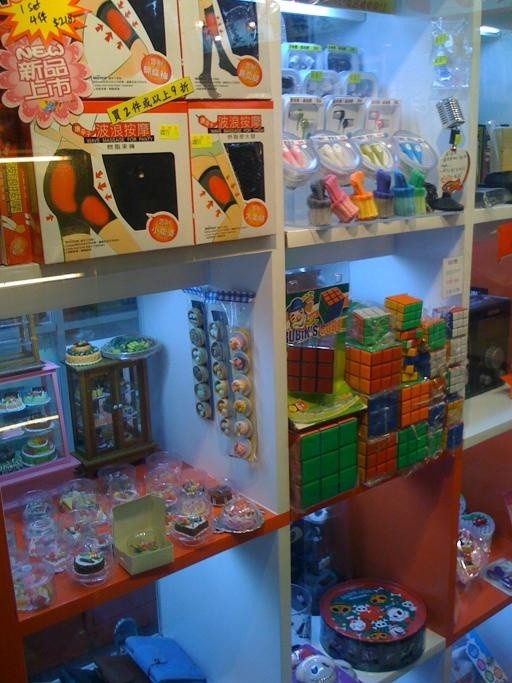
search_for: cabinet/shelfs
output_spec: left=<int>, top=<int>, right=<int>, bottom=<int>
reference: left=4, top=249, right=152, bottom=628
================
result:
left=1, top=0, right=512, bottom=683
